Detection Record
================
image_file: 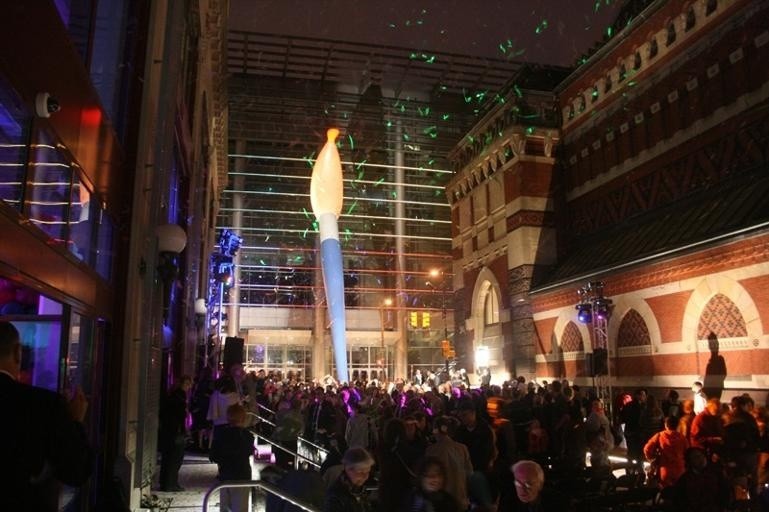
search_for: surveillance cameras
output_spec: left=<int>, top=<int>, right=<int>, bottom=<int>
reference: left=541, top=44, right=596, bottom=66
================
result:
left=36, top=90, right=62, bottom=118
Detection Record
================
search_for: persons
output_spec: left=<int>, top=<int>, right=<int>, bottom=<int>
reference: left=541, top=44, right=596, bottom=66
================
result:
left=159, top=366, right=769, bottom=512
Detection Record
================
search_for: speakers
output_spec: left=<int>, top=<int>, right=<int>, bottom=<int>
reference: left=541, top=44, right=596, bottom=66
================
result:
left=224, top=337, right=244, bottom=373
left=593, top=348, right=608, bottom=374
left=583, top=353, right=593, bottom=376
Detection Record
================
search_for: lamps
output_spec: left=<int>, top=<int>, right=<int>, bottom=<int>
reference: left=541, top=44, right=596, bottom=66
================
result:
left=157, top=223, right=187, bottom=280
left=576, top=281, right=612, bottom=322
left=194, top=299, right=207, bottom=327
left=212, top=229, right=243, bottom=288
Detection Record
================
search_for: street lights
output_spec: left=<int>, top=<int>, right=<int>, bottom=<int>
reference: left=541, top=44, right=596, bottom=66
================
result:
left=575, top=278, right=616, bottom=432
left=425, top=267, right=454, bottom=374
left=204, top=227, right=244, bottom=367
left=378, top=297, right=395, bottom=381
